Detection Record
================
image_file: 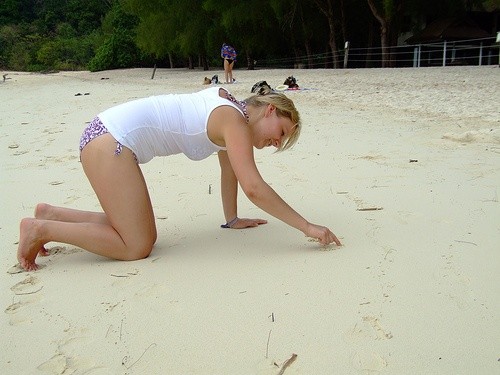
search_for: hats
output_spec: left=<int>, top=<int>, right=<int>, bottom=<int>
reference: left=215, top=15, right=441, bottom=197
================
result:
left=275, top=85, right=288, bottom=90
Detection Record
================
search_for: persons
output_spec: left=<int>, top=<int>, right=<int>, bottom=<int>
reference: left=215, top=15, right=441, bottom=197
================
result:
left=221, top=43, right=236, bottom=83
left=17, top=81, right=342, bottom=270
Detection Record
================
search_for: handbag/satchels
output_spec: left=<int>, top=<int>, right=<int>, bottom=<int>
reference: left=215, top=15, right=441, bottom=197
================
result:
left=251, top=81, right=274, bottom=95
left=204, top=77, right=211, bottom=84
left=283, top=75, right=299, bottom=88
left=212, top=74, right=218, bottom=83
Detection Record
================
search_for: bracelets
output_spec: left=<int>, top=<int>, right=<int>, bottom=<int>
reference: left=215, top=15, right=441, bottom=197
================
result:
left=221, top=216, right=238, bottom=228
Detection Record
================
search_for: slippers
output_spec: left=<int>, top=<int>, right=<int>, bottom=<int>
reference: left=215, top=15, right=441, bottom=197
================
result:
left=288, top=87, right=301, bottom=89
